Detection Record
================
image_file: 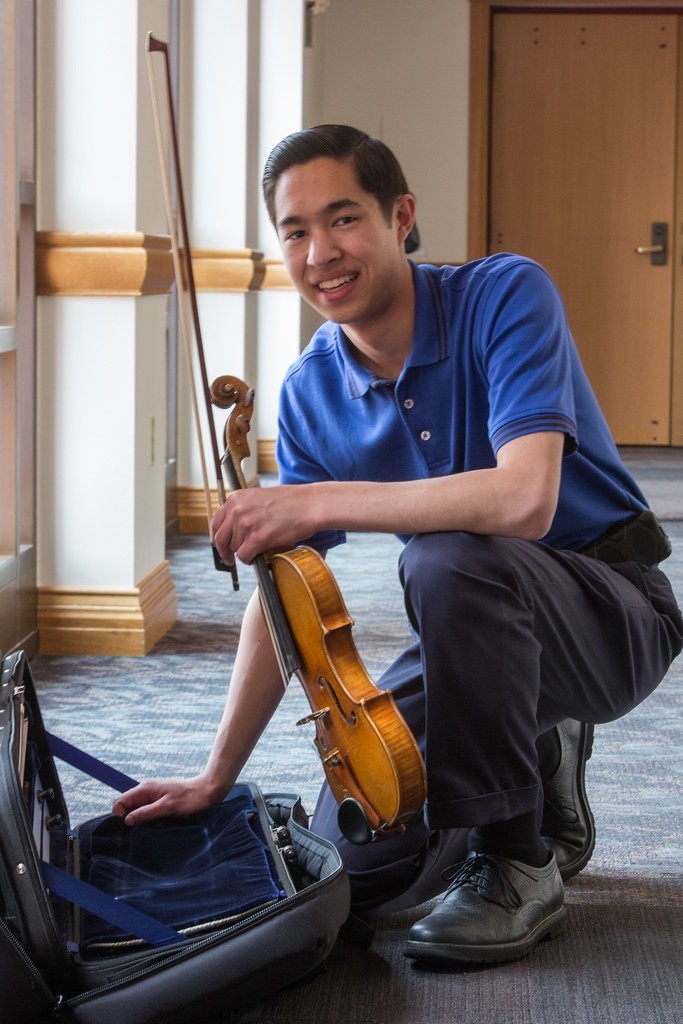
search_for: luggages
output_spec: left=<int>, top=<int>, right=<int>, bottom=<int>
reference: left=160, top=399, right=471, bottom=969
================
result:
left=0, top=650, right=349, bottom=1024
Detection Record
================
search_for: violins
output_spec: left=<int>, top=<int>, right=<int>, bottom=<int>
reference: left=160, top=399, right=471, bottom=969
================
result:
left=203, top=372, right=429, bottom=845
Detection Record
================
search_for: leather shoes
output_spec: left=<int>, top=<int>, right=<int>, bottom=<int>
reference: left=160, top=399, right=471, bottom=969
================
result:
left=402, top=848, right=569, bottom=964
left=537, top=717, right=595, bottom=879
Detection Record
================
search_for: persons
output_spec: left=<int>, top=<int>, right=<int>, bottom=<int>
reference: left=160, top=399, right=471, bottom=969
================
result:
left=113, top=125, right=682, bottom=966
